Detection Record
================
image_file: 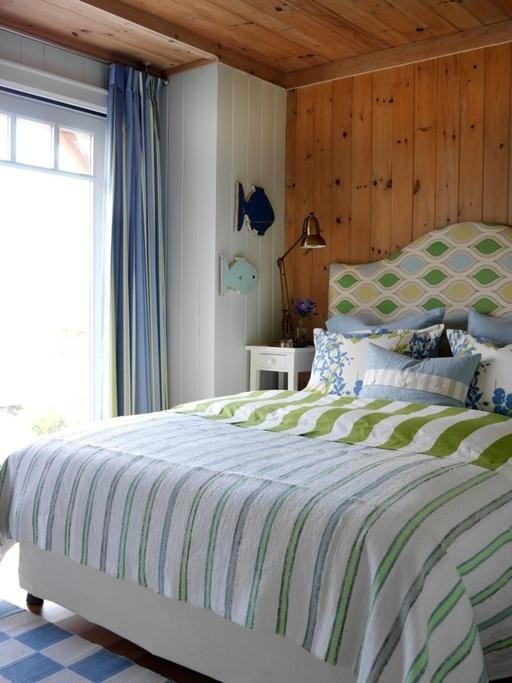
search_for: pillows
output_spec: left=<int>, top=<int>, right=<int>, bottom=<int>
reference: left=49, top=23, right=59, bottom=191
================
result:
left=309, top=313, right=512, bottom=416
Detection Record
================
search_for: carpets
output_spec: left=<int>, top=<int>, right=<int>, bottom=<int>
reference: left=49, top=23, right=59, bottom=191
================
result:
left=0, top=598, right=168, bottom=683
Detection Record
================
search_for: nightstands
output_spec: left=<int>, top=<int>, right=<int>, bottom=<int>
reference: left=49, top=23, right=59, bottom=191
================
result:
left=245, top=345, right=314, bottom=393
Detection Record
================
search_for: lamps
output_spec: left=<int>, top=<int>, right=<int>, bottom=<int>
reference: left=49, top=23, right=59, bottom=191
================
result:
left=271, top=211, right=328, bottom=346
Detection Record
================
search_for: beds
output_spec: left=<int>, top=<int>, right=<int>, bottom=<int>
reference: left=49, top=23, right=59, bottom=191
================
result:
left=0, top=222, right=512, bottom=683
